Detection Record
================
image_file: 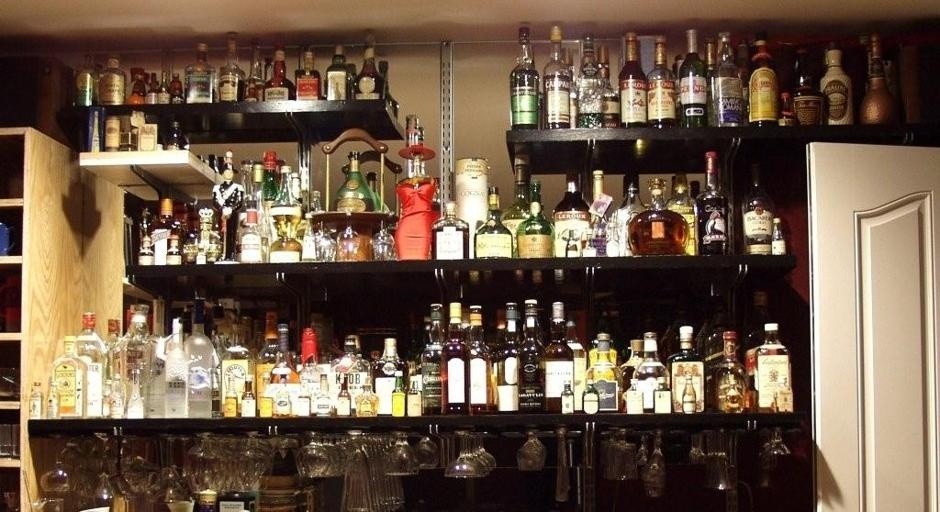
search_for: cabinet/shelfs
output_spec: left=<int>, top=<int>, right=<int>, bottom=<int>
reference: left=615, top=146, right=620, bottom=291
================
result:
left=0, top=126, right=121, bottom=512
left=27, top=95, right=935, bottom=436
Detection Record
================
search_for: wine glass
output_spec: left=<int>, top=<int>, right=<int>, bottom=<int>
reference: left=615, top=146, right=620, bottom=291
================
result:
left=297, top=428, right=572, bottom=512
left=602, top=429, right=792, bottom=498
left=41, top=434, right=274, bottom=504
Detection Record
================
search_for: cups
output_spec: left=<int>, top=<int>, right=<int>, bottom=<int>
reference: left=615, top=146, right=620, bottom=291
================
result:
left=0, top=222, right=16, bottom=256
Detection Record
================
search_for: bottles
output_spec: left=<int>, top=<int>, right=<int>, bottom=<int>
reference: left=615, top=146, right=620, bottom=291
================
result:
left=510, top=27, right=927, bottom=125
left=88, top=107, right=191, bottom=150
left=125, top=152, right=785, bottom=264
left=28, top=297, right=794, bottom=417
left=74, top=35, right=390, bottom=106
